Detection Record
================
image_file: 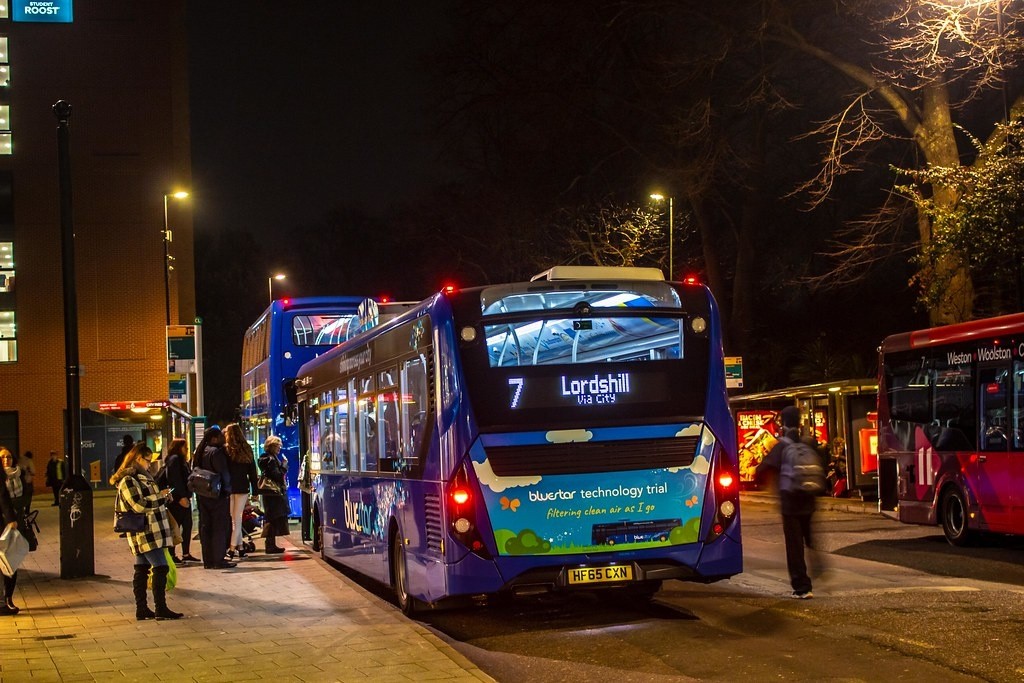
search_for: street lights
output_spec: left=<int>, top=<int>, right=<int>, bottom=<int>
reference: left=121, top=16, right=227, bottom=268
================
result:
left=269, top=274, right=285, bottom=305
left=163, top=188, right=190, bottom=324
left=651, top=193, right=673, bottom=282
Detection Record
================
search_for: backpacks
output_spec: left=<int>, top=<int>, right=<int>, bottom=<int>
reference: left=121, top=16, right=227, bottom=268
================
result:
left=780, top=433, right=825, bottom=494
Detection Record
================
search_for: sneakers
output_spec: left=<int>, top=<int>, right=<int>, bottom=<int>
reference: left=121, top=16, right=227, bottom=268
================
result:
left=791, top=588, right=814, bottom=600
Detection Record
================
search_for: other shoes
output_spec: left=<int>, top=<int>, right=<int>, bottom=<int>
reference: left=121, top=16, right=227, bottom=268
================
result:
left=5, top=597, right=18, bottom=609
left=0, top=605, right=16, bottom=615
left=172, top=557, right=184, bottom=564
left=204, top=563, right=214, bottom=568
left=214, top=561, right=236, bottom=569
left=182, top=555, right=202, bottom=563
left=332, top=541, right=353, bottom=548
left=51, top=501, right=58, bottom=506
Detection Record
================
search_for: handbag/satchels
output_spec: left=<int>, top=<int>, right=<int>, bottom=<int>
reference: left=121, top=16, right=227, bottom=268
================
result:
left=0, top=523, right=29, bottom=577
left=153, top=467, right=169, bottom=506
left=188, top=467, right=221, bottom=498
left=16, top=509, right=38, bottom=552
left=114, top=510, right=146, bottom=532
left=255, top=474, right=282, bottom=497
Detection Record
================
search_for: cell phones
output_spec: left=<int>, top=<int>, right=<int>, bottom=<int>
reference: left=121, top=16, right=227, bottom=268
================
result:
left=165, top=487, right=174, bottom=496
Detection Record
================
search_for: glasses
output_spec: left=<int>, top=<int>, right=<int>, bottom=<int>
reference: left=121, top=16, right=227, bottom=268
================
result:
left=142, top=457, right=151, bottom=463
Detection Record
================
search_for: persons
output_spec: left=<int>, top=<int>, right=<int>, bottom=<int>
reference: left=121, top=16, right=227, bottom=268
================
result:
left=368, top=419, right=392, bottom=454
left=223, top=423, right=259, bottom=560
left=113, top=434, right=133, bottom=532
left=46, top=450, right=64, bottom=508
left=192, top=427, right=237, bottom=569
left=164, top=438, right=202, bottom=564
left=758, top=405, right=831, bottom=598
left=258, top=436, right=291, bottom=554
left=0, top=446, right=26, bottom=615
left=109, top=442, right=185, bottom=620
left=17, top=451, right=36, bottom=514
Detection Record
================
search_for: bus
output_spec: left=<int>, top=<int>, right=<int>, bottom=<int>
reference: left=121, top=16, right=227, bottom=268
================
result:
left=240, top=296, right=397, bottom=522
left=284, top=264, right=745, bottom=612
left=875, top=313, right=1024, bottom=545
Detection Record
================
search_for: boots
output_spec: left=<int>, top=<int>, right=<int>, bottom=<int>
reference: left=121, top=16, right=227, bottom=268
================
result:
left=133, top=564, right=154, bottom=620
left=265, top=539, right=285, bottom=553
left=152, top=565, right=184, bottom=620
